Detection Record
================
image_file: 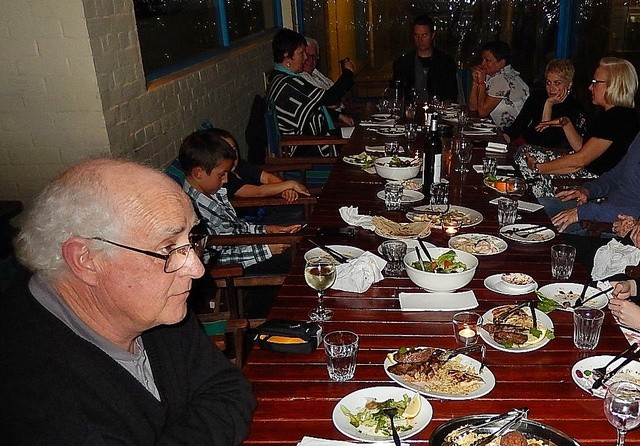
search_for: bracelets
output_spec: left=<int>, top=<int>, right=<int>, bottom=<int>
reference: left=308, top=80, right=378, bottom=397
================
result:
left=478, top=80, right=486, bottom=85
left=533, top=161, right=539, bottom=174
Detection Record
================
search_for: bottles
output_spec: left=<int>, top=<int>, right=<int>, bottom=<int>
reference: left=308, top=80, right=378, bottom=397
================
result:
left=425, top=114, right=442, bottom=184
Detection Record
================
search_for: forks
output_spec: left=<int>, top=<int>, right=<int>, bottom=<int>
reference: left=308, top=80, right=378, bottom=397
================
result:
left=514, top=226, right=553, bottom=238
left=590, top=342, right=638, bottom=378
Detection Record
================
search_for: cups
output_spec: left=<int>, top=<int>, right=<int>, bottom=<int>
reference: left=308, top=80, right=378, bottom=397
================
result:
left=430, top=181, right=449, bottom=205
left=440, top=218, right=462, bottom=236
left=498, top=199, right=517, bottom=224
left=382, top=240, right=407, bottom=275
left=551, top=243, right=576, bottom=279
left=453, top=312, right=483, bottom=346
left=483, top=157, right=497, bottom=177
left=385, top=186, right=403, bottom=209
left=574, top=307, right=605, bottom=349
left=324, top=331, right=359, bottom=382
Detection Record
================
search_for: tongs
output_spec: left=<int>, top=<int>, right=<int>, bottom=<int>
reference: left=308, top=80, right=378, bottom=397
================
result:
left=443, top=406, right=528, bottom=446
left=493, top=299, right=537, bottom=331
left=307, top=239, right=349, bottom=264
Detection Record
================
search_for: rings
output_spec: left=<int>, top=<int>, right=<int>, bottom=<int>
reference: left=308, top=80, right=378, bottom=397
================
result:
left=612, top=226, right=616, bottom=233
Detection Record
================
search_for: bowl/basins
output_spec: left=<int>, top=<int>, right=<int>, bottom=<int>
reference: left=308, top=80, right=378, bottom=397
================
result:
left=404, top=247, right=478, bottom=293
left=374, top=156, right=421, bottom=179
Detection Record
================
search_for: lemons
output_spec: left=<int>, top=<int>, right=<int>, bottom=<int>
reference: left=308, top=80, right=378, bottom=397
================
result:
left=402, top=391, right=421, bottom=419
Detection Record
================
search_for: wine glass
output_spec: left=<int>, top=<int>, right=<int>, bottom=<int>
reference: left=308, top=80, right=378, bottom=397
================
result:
left=410, top=87, right=420, bottom=106
left=506, top=177, right=525, bottom=206
left=305, top=257, right=337, bottom=321
left=458, top=112, right=468, bottom=135
left=385, top=140, right=399, bottom=156
left=405, top=123, right=417, bottom=140
left=604, top=381, right=640, bottom=446
left=454, top=138, right=472, bottom=172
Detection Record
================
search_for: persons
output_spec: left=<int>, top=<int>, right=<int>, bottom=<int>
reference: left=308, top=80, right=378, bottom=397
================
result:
left=468, top=40, right=530, bottom=132
left=179, top=130, right=301, bottom=309
left=536, top=131, right=639, bottom=238
left=512, top=56, right=640, bottom=198
left=557, top=212, right=639, bottom=281
left=264, top=28, right=357, bottom=157
left=501, top=59, right=586, bottom=149
left=206, top=127, right=311, bottom=224
left=0, top=157, right=259, bottom=446
left=376, top=14, right=459, bottom=103
left=593, top=273, right=639, bottom=335
left=298, top=37, right=346, bottom=113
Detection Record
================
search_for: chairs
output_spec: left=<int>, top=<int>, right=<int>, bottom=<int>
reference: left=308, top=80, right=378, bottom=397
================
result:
left=197, top=195, right=317, bottom=315
left=551, top=177, right=618, bottom=237
left=193, top=265, right=248, bottom=370
left=254, top=162, right=323, bottom=214
left=251, top=97, right=350, bottom=166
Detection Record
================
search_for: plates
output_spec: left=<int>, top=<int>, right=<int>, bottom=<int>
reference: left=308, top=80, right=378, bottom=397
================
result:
left=477, top=304, right=554, bottom=353
left=385, top=180, right=423, bottom=190
left=377, top=132, right=404, bottom=137
left=572, top=354, right=640, bottom=399
left=483, top=175, right=528, bottom=191
left=375, top=222, right=431, bottom=238
left=406, top=204, right=483, bottom=228
left=304, top=244, right=366, bottom=268
left=484, top=272, right=538, bottom=294
left=332, top=386, right=433, bottom=442
left=377, top=189, right=424, bottom=204
left=384, top=347, right=495, bottom=399
left=537, top=282, right=609, bottom=311
left=342, top=155, right=370, bottom=165
left=429, top=413, right=581, bottom=446
left=448, top=233, right=508, bottom=255
left=377, top=239, right=437, bottom=259
left=499, top=223, right=550, bottom=242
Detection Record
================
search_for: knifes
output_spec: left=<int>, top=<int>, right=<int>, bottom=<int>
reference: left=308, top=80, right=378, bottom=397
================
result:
left=575, top=279, right=590, bottom=306
left=592, top=351, right=640, bottom=389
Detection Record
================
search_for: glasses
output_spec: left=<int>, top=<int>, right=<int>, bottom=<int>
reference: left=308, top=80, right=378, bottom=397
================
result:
left=86, top=235, right=208, bottom=273
left=591, top=79, right=607, bottom=84
left=307, top=54, right=320, bottom=61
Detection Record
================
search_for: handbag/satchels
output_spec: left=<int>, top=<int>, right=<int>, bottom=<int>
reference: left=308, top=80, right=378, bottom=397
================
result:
left=254, top=319, right=323, bottom=354
